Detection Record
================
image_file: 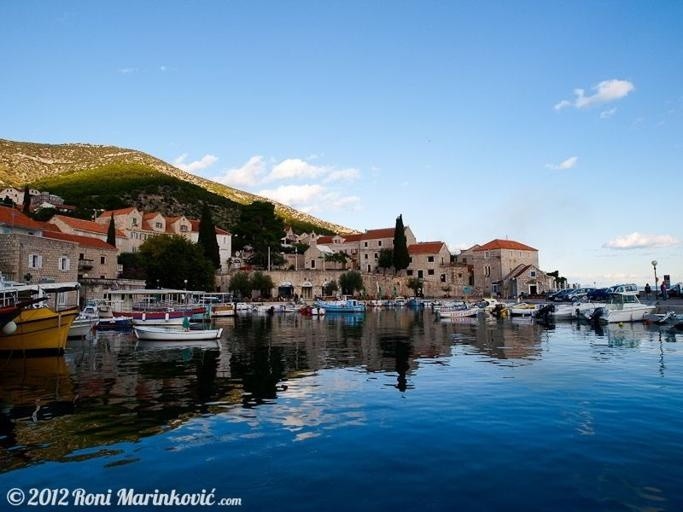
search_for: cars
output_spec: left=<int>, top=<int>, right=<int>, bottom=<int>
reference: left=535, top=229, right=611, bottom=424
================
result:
left=550, top=288, right=608, bottom=300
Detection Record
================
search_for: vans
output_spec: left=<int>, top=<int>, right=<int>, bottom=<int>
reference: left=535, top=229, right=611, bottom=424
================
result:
left=607, top=283, right=640, bottom=298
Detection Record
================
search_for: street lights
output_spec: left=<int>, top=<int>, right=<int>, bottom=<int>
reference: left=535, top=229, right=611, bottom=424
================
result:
left=652, top=260, right=658, bottom=298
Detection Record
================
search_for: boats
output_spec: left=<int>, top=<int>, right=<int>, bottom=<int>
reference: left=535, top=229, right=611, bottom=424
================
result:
left=370, top=296, right=432, bottom=306
left=437, top=296, right=683, bottom=325
left=0, top=278, right=252, bottom=351
left=253, top=298, right=366, bottom=315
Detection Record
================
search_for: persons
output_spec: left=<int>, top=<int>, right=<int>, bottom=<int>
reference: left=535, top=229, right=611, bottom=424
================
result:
left=243, top=293, right=305, bottom=304
left=660, top=282, right=667, bottom=300
left=645, top=284, right=651, bottom=300
